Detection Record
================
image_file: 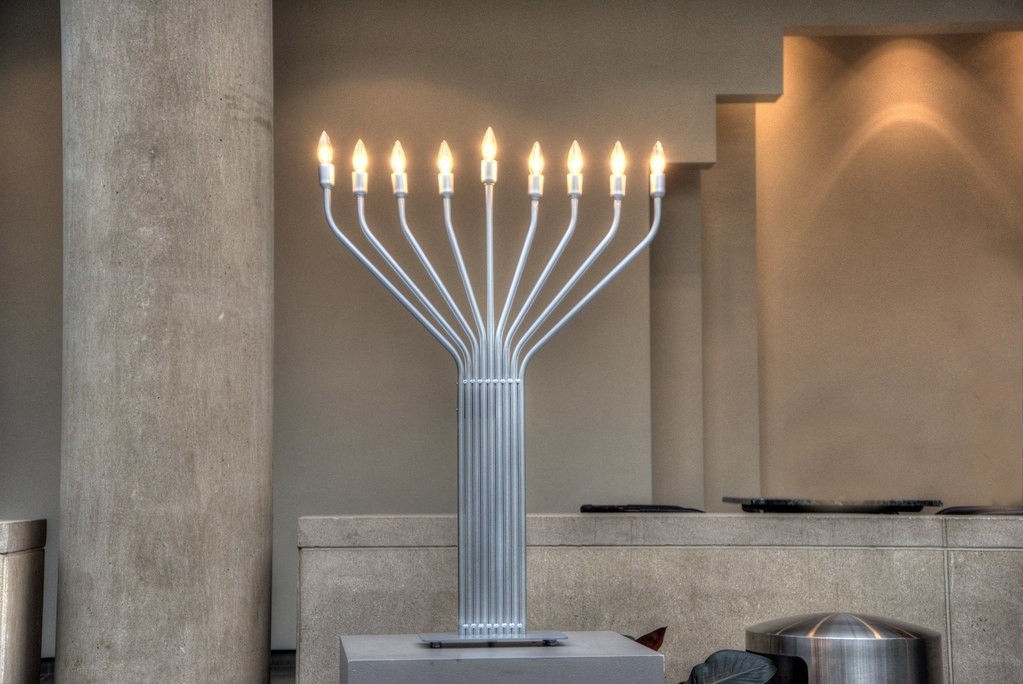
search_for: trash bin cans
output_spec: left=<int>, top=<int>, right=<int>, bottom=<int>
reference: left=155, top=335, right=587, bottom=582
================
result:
left=745, top=610, right=944, bottom=684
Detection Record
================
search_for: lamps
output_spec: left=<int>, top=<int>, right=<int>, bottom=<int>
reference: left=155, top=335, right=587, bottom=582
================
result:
left=317, top=127, right=666, bottom=649
